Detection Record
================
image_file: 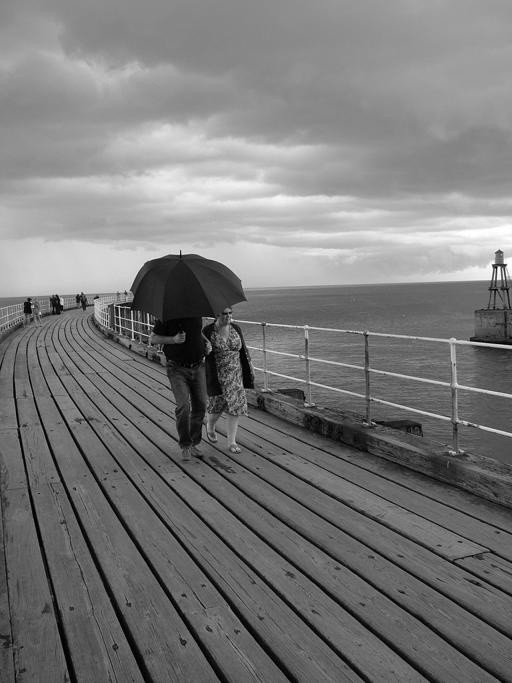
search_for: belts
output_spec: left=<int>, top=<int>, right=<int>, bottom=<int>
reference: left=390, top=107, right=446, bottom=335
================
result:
left=168, top=359, right=199, bottom=368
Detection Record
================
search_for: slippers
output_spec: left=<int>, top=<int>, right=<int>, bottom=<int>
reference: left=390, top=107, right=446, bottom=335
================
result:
left=229, top=444, right=241, bottom=454
left=205, top=423, right=217, bottom=443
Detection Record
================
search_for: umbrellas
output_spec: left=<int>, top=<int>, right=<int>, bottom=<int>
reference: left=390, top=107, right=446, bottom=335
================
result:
left=129, top=249, right=249, bottom=325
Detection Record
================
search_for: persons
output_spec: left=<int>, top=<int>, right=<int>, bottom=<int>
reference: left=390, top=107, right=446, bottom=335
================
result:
left=76, top=294, right=81, bottom=309
left=23, top=298, right=32, bottom=328
left=49, top=293, right=64, bottom=314
left=24, top=296, right=43, bottom=328
left=124, top=290, right=127, bottom=301
left=202, top=306, right=256, bottom=455
left=149, top=316, right=212, bottom=459
left=117, top=291, right=121, bottom=301
left=80, top=292, right=87, bottom=310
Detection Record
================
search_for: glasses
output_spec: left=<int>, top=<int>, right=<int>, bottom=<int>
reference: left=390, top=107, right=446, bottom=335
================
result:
left=222, top=311, right=232, bottom=315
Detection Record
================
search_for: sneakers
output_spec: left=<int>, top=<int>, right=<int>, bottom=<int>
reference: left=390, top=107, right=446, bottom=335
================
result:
left=180, top=444, right=203, bottom=461
